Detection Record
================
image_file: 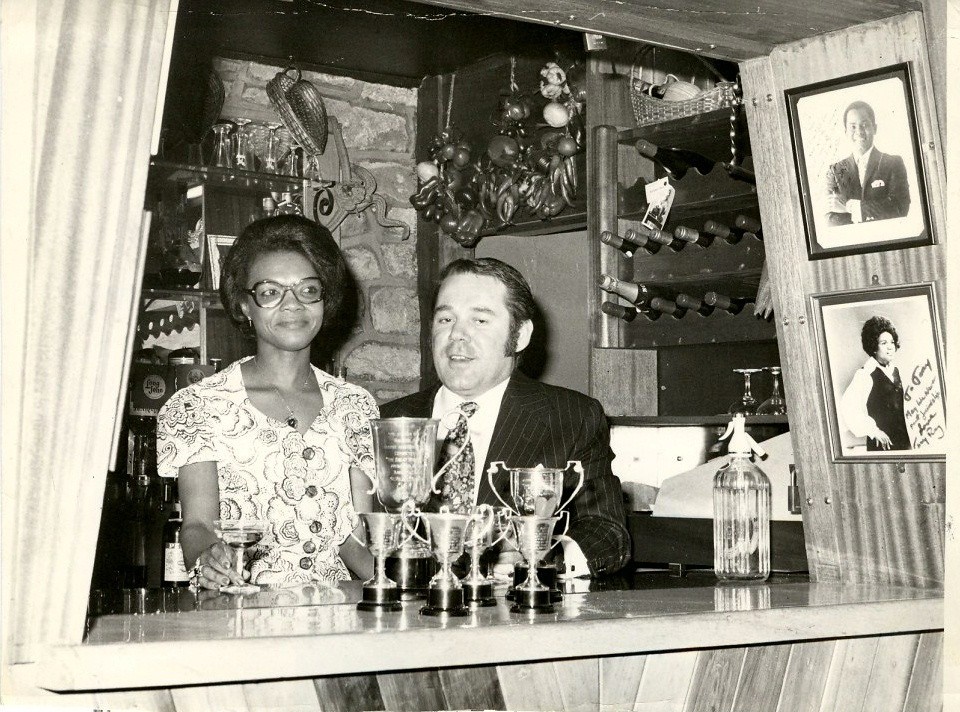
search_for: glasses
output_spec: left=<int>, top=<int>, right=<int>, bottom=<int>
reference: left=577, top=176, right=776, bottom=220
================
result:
left=243, top=276, right=325, bottom=308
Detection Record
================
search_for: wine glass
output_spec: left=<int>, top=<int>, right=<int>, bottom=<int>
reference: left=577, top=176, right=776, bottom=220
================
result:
left=213, top=519, right=270, bottom=595
left=728, top=369, right=762, bottom=415
left=209, top=123, right=233, bottom=170
left=756, top=367, right=786, bottom=415
left=232, top=118, right=255, bottom=172
left=287, top=146, right=299, bottom=177
left=258, top=122, right=283, bottom=175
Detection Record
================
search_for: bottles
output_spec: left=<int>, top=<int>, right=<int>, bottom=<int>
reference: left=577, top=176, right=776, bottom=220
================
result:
left=598, top=274, right=677, bottom=313
left=649, top=228, right=688, bottom=252
left=635, top=139, right=715, bottom=180
left=625, top=229, right=663, bottom=254
left=710, top=222, right=748, bottom=245
left=704, top=291, right=756, bottom=315
left=143, top=182, right=200, bottom=291
left=674, top=225, right=715, bottom=248
left=676, top=294, right=715, bottom=316
left=602, top=301, right=661, bottom=322
left=600, top=231, right=641, bottom=255
left=735, top=214, right=763, bottom=241
left=162, top=499, right=189, bottom=587
left=651, top=297, right=687, bottom=318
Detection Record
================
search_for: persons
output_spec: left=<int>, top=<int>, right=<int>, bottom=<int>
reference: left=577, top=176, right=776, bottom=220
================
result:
left=842, top=315, right=913, bottom=451
left=826, top=100, right=912, bottom=226
left=159, top=215, right=382, bottom=596
left=379, top=259, right=632, bottom=588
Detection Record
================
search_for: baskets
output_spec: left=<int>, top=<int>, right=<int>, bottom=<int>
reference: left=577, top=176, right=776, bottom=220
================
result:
left=266, top=65, right=328, bottom=157
left=195, top=68, right=226, bottom=144
left=629, top=44, right=739, bottom=127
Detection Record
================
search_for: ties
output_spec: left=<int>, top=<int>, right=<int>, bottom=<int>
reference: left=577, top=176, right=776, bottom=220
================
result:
left=432, top=403, right=477, bottom=515
left=857, top=158, right=866, bottom=186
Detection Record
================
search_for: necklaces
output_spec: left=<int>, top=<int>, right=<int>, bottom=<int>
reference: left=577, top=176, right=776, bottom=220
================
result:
left=252, top=356, right=312, bottom=427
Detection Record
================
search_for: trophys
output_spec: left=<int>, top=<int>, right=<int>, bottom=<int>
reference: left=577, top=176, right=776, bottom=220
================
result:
left=485, top=460, right=584, bottom=614
left=347, top=410, right=512, bottom=617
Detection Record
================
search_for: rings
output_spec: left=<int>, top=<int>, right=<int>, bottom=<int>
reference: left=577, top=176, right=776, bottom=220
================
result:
left=199, top=565, right=205, bottom=576
